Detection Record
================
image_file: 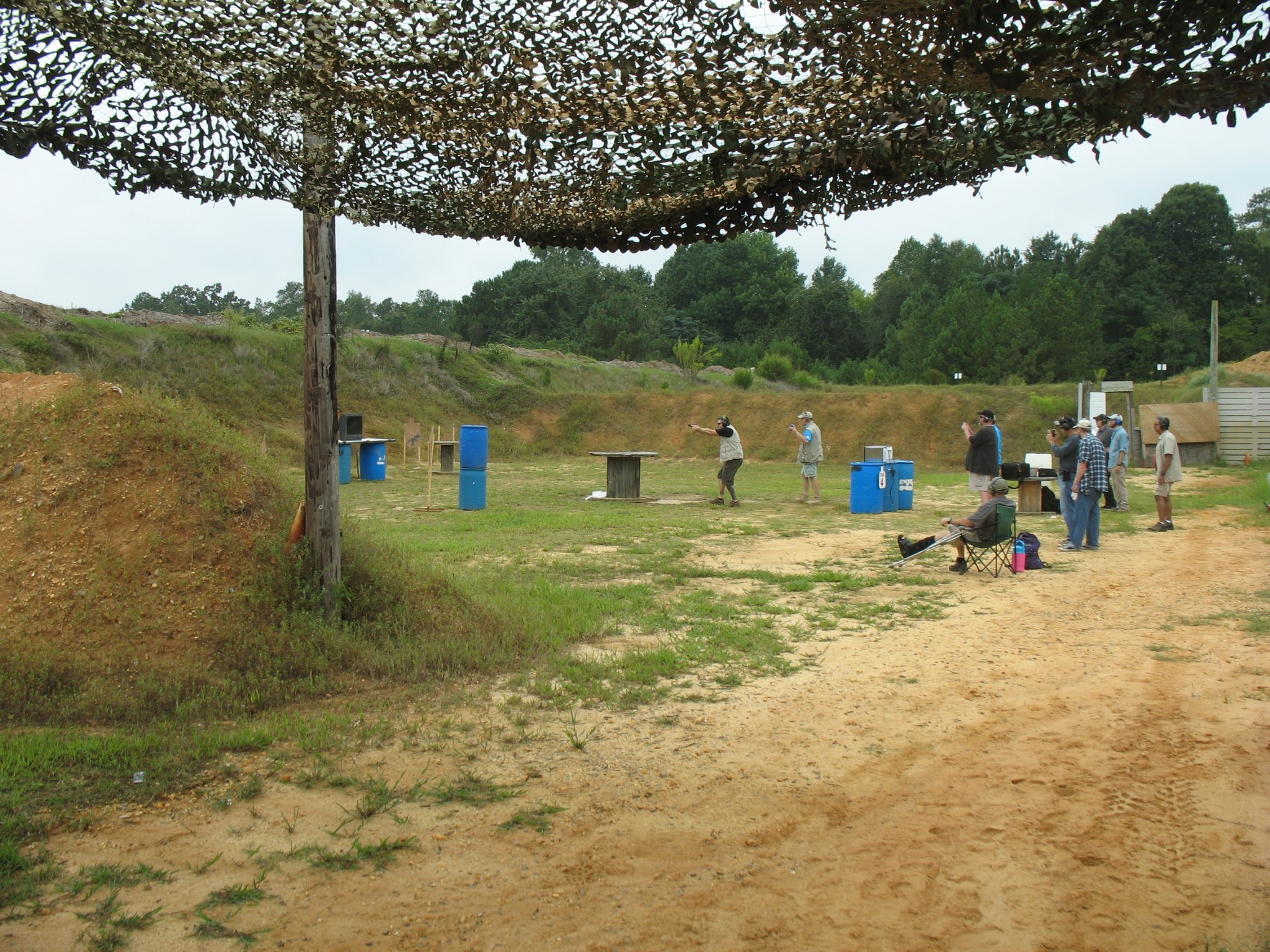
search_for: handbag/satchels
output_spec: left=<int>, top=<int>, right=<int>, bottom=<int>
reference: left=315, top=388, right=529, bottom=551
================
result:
left=1037, top=468, right=1057, bottom=477
left=1001, top=463, right=1030, bottom=489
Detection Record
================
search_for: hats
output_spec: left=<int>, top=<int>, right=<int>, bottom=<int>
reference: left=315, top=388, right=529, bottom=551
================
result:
left=797, top=411, right=812, bottom=418
left=1054, top=415, right=1077, bottom=429
left=1093, top=414, right=1107, bottom=420
left=987, top=477, right=1008, bottom=494
left=1107, top=414, right=1123, bottom=422
left=977, top=409, right=994, bottom=419
left=1072, top=418, right=1092, bottom=430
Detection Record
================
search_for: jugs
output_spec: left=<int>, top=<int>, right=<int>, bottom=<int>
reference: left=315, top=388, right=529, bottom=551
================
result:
left=1015, top=540, right=1025, bottom=553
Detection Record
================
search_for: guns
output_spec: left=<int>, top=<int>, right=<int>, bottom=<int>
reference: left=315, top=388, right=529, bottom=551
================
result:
left=688, top=424, right=698, bottom=427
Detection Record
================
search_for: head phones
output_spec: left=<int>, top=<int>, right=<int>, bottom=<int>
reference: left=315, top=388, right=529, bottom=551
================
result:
left=988, top=478, right=1009, bottom=495
left=804, top=412, right=810, bottom=421
left=1161, top=416, right=1166, bottom=428
left=1061, top=415, right=1069, bottom=430
left=1102, top=414, right=1107, bottom=424
left=984, top=409, right=990, bottom=422
left=723, top=415, right=728, bottom=427
left=1116, top=414, right=1121, bottom=424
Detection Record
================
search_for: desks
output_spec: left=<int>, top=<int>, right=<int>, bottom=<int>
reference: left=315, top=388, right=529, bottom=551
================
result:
left=1006, top=477, right=1058, bottom=516
left=427, top=441, right=461, bottom=474
left=592, top=452, right=659, bottom=502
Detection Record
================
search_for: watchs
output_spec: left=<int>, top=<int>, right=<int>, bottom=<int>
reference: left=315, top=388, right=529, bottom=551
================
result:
left=1160, top=471, right=1165, bottom=476
left=947, top=517, right=952, bottom=524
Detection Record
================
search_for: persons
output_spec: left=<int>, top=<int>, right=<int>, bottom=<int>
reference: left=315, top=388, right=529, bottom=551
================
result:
left=1147, top=415, right=1181, bottom=533
left=1109, top=413, right=1129, bottom=514
left=1046, top=415, right=1081, bottom=547
left=789, top=411, right=823, bottom=507
left=898, top=475, right=1015, bottom=573
left=689, top=415, right=744, bottom=507
left=1057, top=419, right=1109, bottom=553
left=961, top=409, right=1002, bottom=505
left=1093, top=413, right=1116, bottom=510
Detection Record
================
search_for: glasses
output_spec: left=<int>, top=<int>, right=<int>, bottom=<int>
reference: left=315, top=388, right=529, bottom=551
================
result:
left=717, top=422, right=723, bottom=425
left=1154, top=423, right=1161, bottom=426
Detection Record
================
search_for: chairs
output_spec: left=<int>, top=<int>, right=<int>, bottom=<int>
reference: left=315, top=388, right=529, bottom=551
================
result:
left=946, top=504, right=1017, bottom=577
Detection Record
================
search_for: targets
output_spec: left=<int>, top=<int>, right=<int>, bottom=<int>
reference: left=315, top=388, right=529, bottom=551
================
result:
left=404, top=417, right=421, bottom=447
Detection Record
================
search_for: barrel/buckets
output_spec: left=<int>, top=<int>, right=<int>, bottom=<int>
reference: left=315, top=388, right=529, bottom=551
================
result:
left=339, top=443, right=351, bottom=484
left=459, top=468, right=487, bottom=510
left=893, top=460, right=914, bottom=509
left=1012, top=553, right=1025, bottom=572
left=459, top=425, right=488, bottom=468
left=360, top=442, right=386, bottom=481
left=850, top=462, right=884, bottom=514
left=866, top=461, right=899, bottom=512
left=1030, top=467, right=1038, bottom=476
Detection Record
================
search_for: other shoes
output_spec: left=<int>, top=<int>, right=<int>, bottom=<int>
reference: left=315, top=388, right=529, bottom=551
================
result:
left=806, top=496, right=823, bottom=505
left=1165, top=520, right=1174, bottom=530
left=1058, top=540, right=1071, bottom=543
left=897, top=535, right=911, bottom=558
left=728, top=501, right=740, bottom=507
left=1148, top=522, right=1168, bottom=532
left=1057, top=543, right=1068, bottom=546
left=1100, top=506, right=1111, bottom=510
left=949, top=560, right=969, bottom=572
left=795, top=493, right=809, bottom=502
left=707, top=497, right=724, bottom=505
left=1113, top=507, right=1126, bottom=512
left=1082, top=545, right=1098, bottom=550
left=1058, top=546, right=1081, bottom=551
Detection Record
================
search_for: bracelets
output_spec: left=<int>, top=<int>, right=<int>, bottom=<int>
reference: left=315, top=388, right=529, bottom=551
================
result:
left=1116, top=463, right=1126, bottom=467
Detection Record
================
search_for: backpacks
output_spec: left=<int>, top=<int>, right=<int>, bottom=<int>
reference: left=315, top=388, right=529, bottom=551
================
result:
left=1041, top=486, right=1061, bottom=514
left=1017, top=532, right=1044, bottom=570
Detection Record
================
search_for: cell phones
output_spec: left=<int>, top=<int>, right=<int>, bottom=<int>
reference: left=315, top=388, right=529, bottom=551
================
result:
left=1050, top=430, right=1055, bottom=437
